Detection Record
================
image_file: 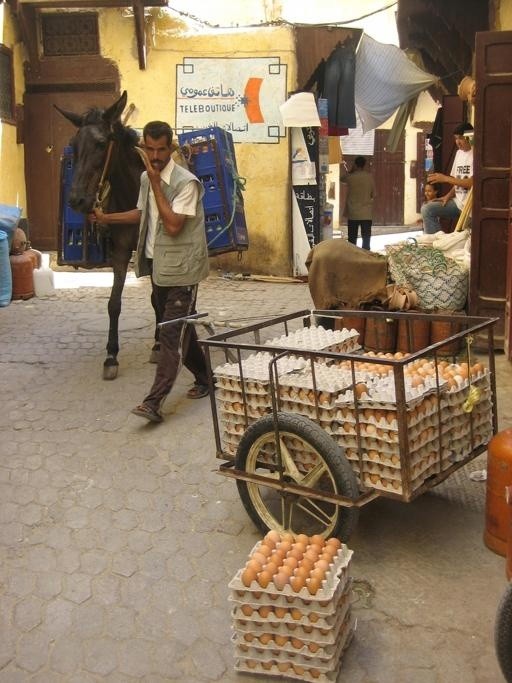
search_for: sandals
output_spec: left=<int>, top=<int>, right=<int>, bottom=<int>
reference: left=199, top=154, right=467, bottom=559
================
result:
left=131, top=405, right=162, bottom=423
left=186, top=378, right=217, bottom=400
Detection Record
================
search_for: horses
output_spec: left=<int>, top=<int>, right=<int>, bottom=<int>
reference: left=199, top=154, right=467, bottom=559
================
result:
left=51, top=90, right=161, bottom=381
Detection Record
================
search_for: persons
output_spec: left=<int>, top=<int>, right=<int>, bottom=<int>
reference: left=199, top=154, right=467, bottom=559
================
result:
left=89, top=120, right=218, bottom=423
left=345, top=156, right=375, bottom=251
left=416, top=183, right=442, bottom=232
left=421, top=123, right=473, bottom=233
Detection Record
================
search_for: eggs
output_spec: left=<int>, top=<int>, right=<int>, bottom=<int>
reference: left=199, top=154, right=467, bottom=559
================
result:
left=212, top=325, right=494, bottom=495
left=228, top=530, right=355, bottom=683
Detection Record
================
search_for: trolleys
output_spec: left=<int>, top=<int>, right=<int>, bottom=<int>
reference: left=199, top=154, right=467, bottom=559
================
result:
left=155, top=308, right=498, bottom=545
